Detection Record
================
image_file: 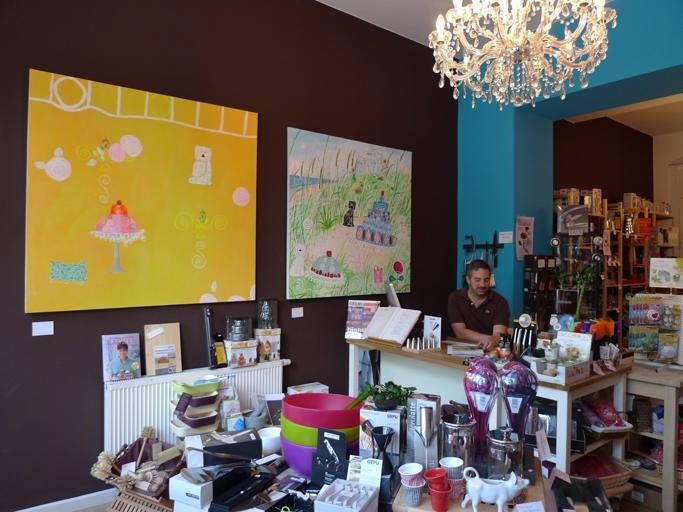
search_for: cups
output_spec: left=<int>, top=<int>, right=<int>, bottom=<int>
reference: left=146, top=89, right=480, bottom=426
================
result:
left=423, top=468, right=449, bottom=490
left=449, top=478, right=463, bottom=500
left=398, top=463, right=423, bottom=486
left=401, top=480, right=426, bottom=506
left=427, top=484, right=452, bottom=511
left=439, top=457, right=464, bottom=479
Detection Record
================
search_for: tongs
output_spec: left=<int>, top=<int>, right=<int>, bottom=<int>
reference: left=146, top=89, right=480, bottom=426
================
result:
left=181, top=447, right=254, bottom=484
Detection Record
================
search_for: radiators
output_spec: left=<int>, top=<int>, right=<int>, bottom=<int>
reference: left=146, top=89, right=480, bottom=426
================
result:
left=103, top=359, right=289, bottom=458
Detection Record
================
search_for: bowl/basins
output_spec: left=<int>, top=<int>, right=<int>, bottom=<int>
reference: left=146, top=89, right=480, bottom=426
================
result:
left=280, top=435, right=360, bottom=477
left=280, top=413, right=360, bottom=447
left=282, top=393, right=365, bottom=428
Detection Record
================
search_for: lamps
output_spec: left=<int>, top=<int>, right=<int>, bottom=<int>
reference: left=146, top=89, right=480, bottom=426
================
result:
left=431, top=0, right=616, bottom=111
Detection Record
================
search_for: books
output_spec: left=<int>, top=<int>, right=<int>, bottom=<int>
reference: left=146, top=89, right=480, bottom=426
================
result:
left=363, top=306, right=422, bottom=345
left=344, top=300, right=380, bottom=340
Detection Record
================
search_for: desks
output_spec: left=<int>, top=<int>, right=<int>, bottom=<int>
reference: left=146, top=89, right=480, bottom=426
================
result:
left=349, top=337, right=631, bottom=478
left=617, top=354, right=683, bottom=512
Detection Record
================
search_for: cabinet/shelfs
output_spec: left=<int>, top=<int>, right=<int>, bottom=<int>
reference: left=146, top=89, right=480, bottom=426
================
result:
left=556, top=188, right=678, bottom=324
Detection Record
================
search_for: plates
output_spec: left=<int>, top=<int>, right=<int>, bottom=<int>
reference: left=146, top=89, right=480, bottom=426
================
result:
left=170, top=400, right=218, bottom=415
left=175, top=412, right=217, bottom=428
left=172, top=374, right=218, bottom=395
left=171, top=420, right=219, bottom=436
left=177, top=390, right=218, bottom=407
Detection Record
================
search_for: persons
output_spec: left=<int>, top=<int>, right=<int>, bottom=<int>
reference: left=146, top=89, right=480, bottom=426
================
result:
left=102, top=334, right=141, bottom=381
left=448, top=259, right=510, bottom=351
